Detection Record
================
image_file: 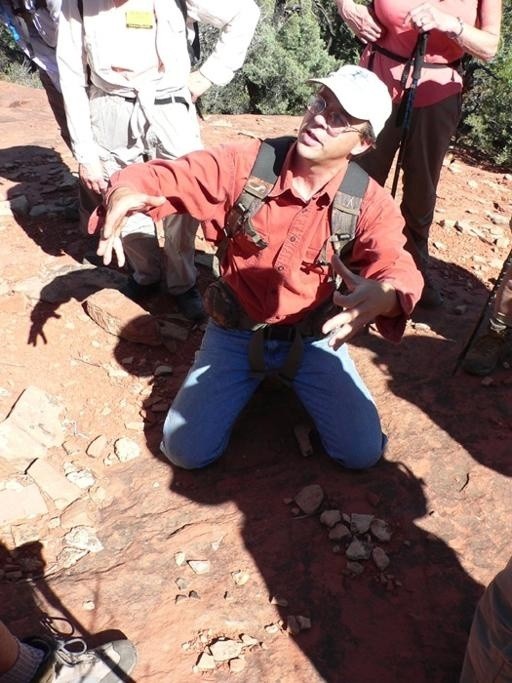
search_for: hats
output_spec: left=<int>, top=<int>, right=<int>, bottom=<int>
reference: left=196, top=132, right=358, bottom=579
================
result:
left=307, top=64, right=393, bottom=139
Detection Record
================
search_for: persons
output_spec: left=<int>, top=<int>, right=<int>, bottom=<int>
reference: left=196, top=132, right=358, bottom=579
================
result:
left=1, top=619, right=137, bottom=683
left=459, top=557, right=512, bottom=683
left=55, top=1, right=262, bottom=320
left=0, top=0, right=104, bottom=248
left=460, top=219, right=511, bottom=375
left=87, top=64, right=426, bottom=471
left=332, top=0, right=503, bottom=277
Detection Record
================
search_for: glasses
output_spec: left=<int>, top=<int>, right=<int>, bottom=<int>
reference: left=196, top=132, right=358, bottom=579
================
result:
left=306, top=95, right=366, bottom=135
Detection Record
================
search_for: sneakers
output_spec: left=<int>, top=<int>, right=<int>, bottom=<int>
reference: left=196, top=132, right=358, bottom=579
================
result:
left=120, top=274, right=207, bottom=319
left=21, top=634, right=137, bottom=682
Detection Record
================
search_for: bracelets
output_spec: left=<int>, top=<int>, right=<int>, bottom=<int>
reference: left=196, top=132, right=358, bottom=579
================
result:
left=447, top=16, right=465, bottom=40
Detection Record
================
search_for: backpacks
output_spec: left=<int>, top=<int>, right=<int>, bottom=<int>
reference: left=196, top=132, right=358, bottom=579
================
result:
left=202, top=282, right=235, bottom=329
